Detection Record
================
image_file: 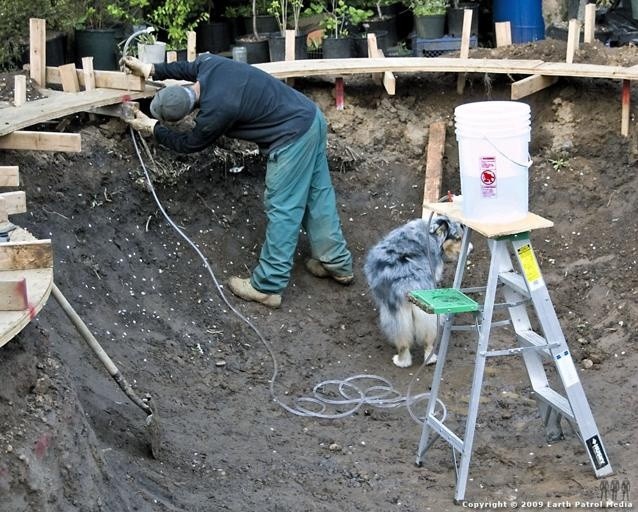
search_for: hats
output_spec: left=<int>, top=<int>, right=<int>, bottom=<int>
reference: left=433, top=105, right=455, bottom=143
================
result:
left=149, top=85, right=196, bottom=124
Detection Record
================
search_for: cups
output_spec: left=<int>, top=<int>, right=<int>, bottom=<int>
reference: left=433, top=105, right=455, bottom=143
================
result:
left=232, top=46, right=247, bottom=66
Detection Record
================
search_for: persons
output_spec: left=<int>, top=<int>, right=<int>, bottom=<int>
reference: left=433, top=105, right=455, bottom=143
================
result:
left=116, top=50, right=356, bottom=311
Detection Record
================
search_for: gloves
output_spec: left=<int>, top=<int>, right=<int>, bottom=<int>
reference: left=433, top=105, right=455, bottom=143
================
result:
left=121, top=107, right=159, bottom=138
left=119, top=56, right=153, bottom=81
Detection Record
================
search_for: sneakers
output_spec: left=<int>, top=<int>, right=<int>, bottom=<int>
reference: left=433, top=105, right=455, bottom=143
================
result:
left=306, top=258, right=354, bottom=283
left=228, top=277, right=282, bottom=308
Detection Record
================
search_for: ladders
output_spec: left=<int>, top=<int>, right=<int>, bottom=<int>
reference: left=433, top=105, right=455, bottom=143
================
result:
left=415, top=226, right=615, bottom=506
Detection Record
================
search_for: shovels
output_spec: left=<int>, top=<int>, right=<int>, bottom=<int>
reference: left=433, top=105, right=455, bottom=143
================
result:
left=52, top=281, right=160, bottom=459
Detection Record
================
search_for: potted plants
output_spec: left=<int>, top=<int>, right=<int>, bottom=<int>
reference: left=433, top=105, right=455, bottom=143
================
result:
left=0, top=0, right=617, bottom=76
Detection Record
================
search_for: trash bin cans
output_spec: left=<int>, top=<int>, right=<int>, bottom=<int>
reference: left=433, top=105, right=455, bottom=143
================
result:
left=493, top=0, right=545, bottom=47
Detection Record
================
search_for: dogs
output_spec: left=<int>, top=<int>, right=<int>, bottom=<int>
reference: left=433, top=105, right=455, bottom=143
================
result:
left=362, top=215, right=474, bottom=369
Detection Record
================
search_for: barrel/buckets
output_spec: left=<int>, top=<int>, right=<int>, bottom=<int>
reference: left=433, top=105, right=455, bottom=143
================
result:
left=498, top=1, right=545, bottom=43
left=455, top=101, right=534, bottom=224
